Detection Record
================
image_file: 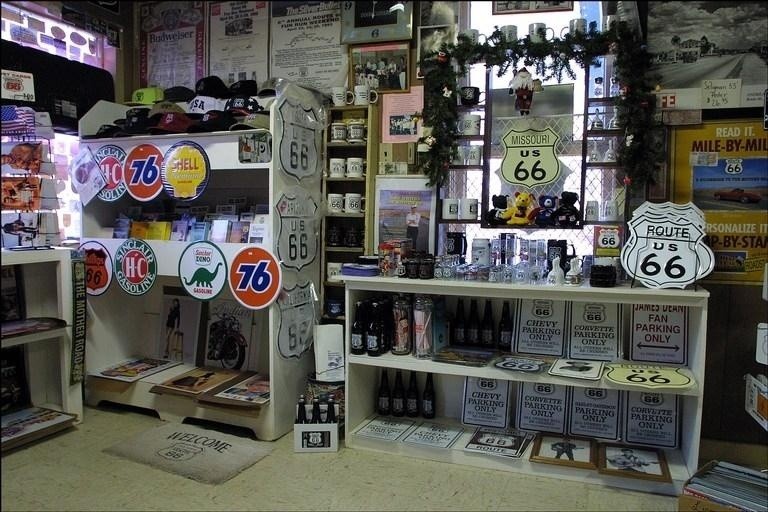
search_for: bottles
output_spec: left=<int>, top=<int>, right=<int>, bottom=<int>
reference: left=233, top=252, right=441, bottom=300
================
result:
left=589, top=140, right=616, bottom=163
left=609, top=77, right=619, bottom=98
left=592, top=109, right=603, bottom=131
left=608, top=108, right=623, bottom=130
left=453, top=297, right=465, bottom=345
left=482, top=299, right=494, bottom=344
left=586, top=201, right=599, bottom=220
left=465, top=298, right=481, bottom=347
left=351, top=292, right=432, bottom=359
left=294, top=393, right=335, bottom=447
left=376, top=369, right=434, bottom=417
left=378, top=237, right=434, bottom=280
left=594, top=77, right=603, bottom=99
left=500, top=299, right=512, bottom=348
left=433, top=232, right=591, bottom=286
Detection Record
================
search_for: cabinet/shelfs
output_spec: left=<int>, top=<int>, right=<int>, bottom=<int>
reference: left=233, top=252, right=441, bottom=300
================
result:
left=583, top=48, right=628, bottom=223
left=436, top=56, right=490, bottom=222
left=0, top=250, right=76, bottom=458
left=344, top=275, right=706, bottom=495
left=323, top=105, right=374, bottom=330
left=2, top=128, right=64, bottom=251
left=77, top=84, right=324, bottom=443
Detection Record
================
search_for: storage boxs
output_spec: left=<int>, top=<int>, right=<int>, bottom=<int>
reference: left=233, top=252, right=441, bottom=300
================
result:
left=292, top=403, right=340, bottom=454
left=676, top=459, right=767, bottom=511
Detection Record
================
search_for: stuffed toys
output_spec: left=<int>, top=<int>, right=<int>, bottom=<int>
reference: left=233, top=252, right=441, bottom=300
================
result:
left=484, top=191, right=579, bottom=228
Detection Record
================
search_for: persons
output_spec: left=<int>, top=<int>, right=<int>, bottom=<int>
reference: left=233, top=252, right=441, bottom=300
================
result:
left=507, top=68, right=535, bottom=115
left=403, top=204, right=421, bottom=251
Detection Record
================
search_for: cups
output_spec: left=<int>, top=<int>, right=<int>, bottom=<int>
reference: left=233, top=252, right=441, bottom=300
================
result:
left=560, top=20, right=588, bottom=41
left=602, top=14, right=619, bottom=35
left=328, top=220, right=364, bottom=247
left=493, top=25, right=518, bottom=45
left=326, top=262, right=356, bottom=284
left=332, top=85, right=379, bottom=105
left=331, top=121, right=367, bottom=142
left=457, top=115, right=481, bottom=136
left=604, top=201, right=620, bottom=221
left=324, top=297, right=341, bottom=314
left=461, top=87, right=486, bottom=106
left=460, top=199, right=481, bottom=219
left=467, top=146, right=481, bottom=165
left=440, top=198, right=459, bottom=219
left=327, top=192, right=365, bottom=214
left=529, top=23, right=555, bottom=44
left=329, top=157, right=367, bottom=178
left=450, top=147, right=466, bottom=165
left=460, top=30, right=488, bottom=46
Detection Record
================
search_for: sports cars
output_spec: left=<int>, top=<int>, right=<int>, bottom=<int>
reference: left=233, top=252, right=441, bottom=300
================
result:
left=713, top=188, right=762, bottom=204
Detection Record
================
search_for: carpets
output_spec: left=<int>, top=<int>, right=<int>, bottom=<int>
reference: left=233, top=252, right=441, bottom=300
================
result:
left=101, top=422, right=276, bottom=487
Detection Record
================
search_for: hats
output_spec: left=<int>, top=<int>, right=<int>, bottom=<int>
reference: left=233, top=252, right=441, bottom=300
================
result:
left=96, top=97, right=270, bottom=137
left=229, top=79, right=256, bottom=98
left=258, top=75, right=286, bottom=94
left=195, top=75, right=230, bottom=98
left=124, top=87, right=163, bottom=106
left=152, top=86, right=194, bottom=104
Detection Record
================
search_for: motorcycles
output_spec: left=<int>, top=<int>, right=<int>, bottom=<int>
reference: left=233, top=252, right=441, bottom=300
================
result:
left=209, top=307, right=247, bottom=372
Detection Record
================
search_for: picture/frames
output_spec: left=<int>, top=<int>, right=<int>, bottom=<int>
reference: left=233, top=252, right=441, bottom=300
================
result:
left=598, top=442, right=674, bottom=485
left=491, top=0, right=573, bottom=15
left=415, top=23, right=453, bottom=79
left=530, top=431, right=599, bottom=471
left=347, top=40, right=410, bottom=94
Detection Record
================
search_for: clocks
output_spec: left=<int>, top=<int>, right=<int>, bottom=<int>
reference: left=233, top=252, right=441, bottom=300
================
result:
left=340, top=1, right=414, bottom=43
